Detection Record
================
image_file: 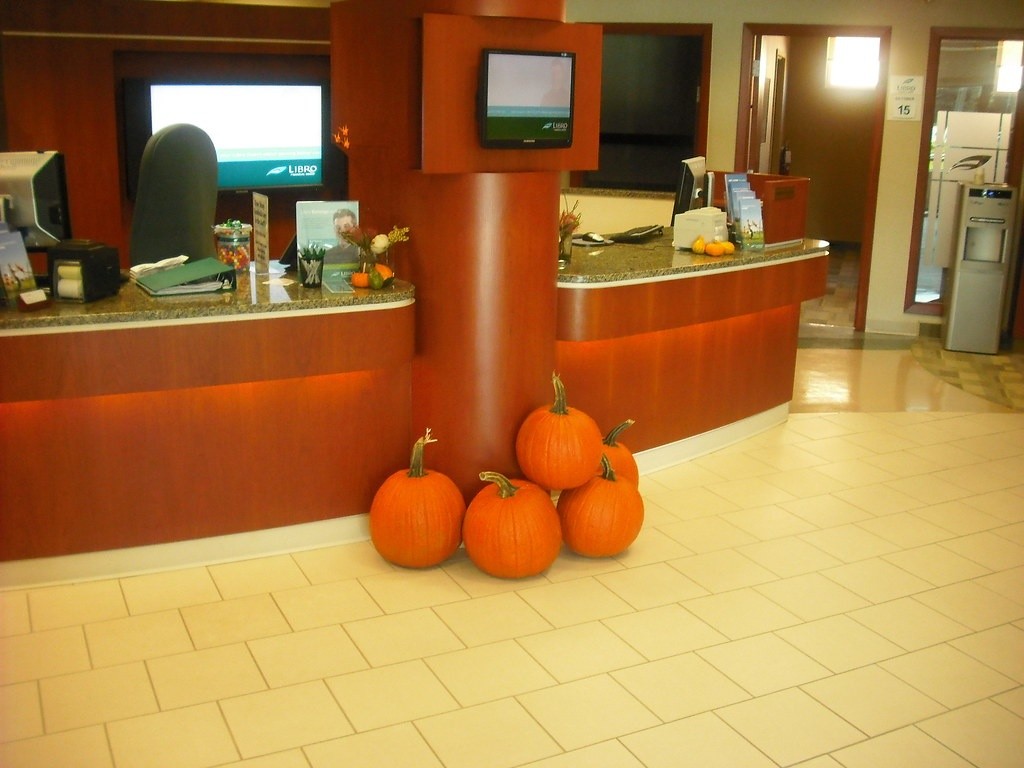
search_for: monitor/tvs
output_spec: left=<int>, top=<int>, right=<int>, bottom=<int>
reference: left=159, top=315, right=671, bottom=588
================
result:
left=475, top=48, right=576, bottom=149
left=122, top=76, right=330, bottom=197
left=279, top=232, right=298, bottom=272
left=0, top=150, right=72, bottom=252
left=671, top=156, right=705, bottom=227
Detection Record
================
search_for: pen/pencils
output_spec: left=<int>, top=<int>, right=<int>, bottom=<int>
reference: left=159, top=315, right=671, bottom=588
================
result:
left=296, top=248, right=325, bottom=285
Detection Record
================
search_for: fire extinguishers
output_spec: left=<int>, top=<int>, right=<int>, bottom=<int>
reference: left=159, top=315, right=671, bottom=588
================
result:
left=779, top=141, right=791, bottom=176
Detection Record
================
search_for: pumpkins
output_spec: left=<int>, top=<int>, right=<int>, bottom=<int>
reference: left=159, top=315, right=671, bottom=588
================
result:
left=369, top=369, right=645, bottom=576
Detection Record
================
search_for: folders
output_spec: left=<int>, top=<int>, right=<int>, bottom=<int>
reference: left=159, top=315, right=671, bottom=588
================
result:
left=135, top=257, right=237, bottom=297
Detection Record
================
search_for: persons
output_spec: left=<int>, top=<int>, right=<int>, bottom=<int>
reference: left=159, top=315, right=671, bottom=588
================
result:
left=747, top=218, right=754, bottom=238
left=323, top=208, right=360, bottom=264
left=541, top=59, right=570, bottom=107
left=8, top=263, right=24, bottom=290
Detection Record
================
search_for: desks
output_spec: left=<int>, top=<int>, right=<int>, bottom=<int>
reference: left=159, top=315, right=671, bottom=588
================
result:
left=0, top=260, right=414, bottom=561
left=556, top=227, right=830, bottom=453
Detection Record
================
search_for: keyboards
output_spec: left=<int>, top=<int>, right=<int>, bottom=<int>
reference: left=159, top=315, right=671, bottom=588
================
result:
left=609, top=225, right=664, bottom=243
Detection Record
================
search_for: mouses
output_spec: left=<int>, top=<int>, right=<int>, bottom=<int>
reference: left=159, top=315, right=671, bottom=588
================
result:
left=582, top=232, right=604, bottom=243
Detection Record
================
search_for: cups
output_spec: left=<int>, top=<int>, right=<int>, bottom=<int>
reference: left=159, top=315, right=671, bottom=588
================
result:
left=973, top=167, right=987, bottom=186
left=557, top=230, right=575, bottom=269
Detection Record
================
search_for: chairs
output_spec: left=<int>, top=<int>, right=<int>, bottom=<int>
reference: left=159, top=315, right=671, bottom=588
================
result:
left=131, top=125, right=218, bottom=266
